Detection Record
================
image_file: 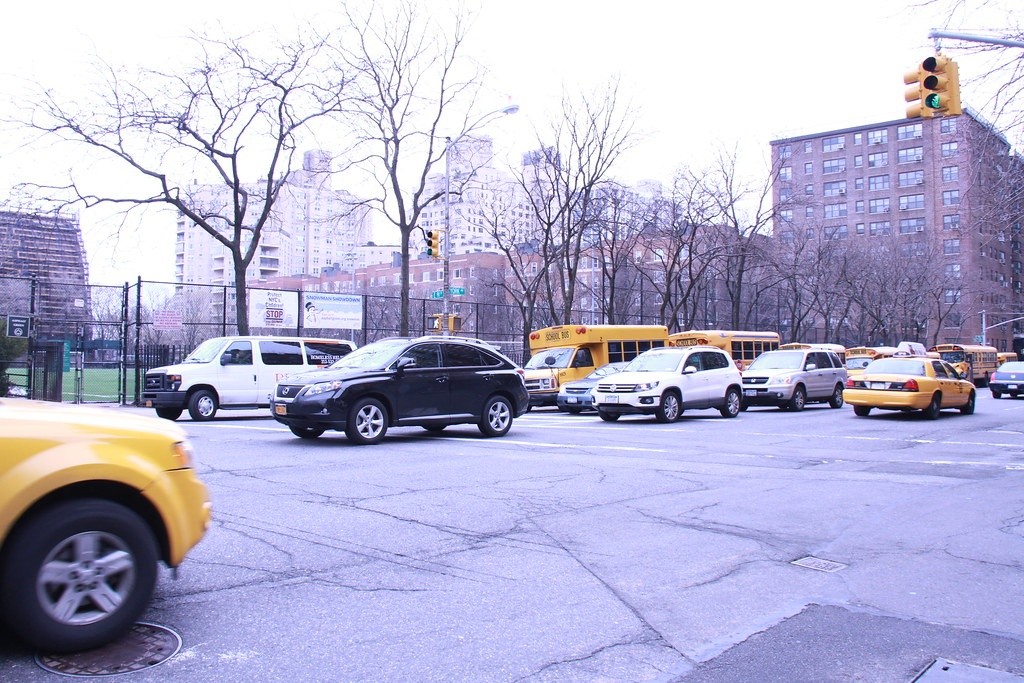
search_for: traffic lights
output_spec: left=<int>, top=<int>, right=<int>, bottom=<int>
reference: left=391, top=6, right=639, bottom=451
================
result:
left=450, top=316, right=462, bottom=331
left=904, top=65, right=924, bottom=121
left=919, top=55, right=957, bottom=118
left=425, top=316, right=444, bottom=333
left=427, top=230, right=439, bottom=258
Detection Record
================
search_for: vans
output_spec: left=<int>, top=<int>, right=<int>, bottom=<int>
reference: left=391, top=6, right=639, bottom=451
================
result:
left=141, top=336, right=358, bottom=423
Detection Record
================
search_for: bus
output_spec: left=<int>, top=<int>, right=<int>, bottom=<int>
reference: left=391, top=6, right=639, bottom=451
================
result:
left=519, top=322, right=669, bottom=413
left=779, top=341, right=846, bottom=368
left=669, top=329, right=780, bottom=376
left=995, top=353, right=1017, bottom=366
left=929, top=343, right=998, bottom=388
left=843, top=346, right=942, bottom=378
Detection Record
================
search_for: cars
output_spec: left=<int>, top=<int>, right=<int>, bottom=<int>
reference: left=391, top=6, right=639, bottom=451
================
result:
left=987, top=361, right=1024, bottom=399
left=842, top=342, right=977, bottom=421
left=557, top=362, right=632, bottom=415
left=0, top=396, right=212, bottom=658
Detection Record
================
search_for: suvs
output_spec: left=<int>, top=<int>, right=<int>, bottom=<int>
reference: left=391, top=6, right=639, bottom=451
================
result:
left=591, top=345, right=744, bottom=423
left=270, top=335, right=531, bottom=446
left=738, top=349, right=847, bottom=412
